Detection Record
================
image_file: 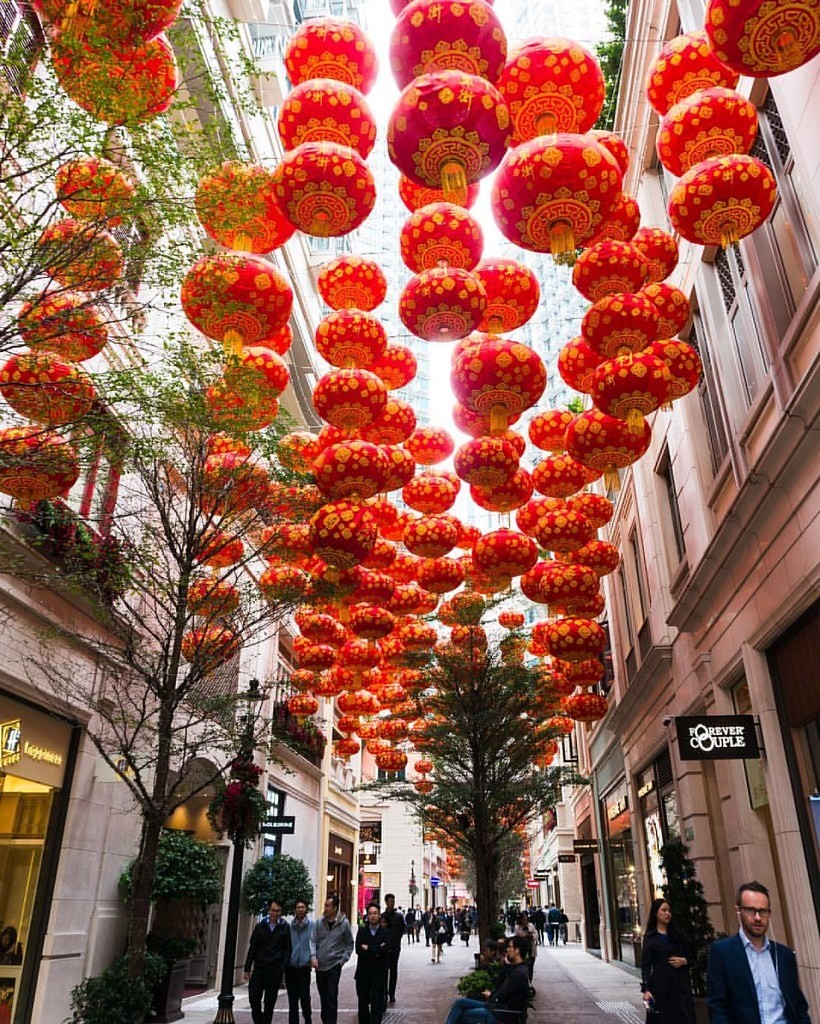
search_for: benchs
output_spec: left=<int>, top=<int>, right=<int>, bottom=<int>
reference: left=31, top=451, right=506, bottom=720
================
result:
left=486, top=984, right=537, bottom=1024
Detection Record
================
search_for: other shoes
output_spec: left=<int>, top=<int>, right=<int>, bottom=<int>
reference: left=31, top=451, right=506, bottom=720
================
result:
left=431, top=958, right=435, bottom=963
left=389, top=995, right=395, bottom=1003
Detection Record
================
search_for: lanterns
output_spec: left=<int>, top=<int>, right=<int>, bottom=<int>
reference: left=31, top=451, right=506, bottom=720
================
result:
left=182, top=158, right=297, bottom=362
left=313, top=258, right=386, bottom=600
left=490, top=40, right=627, bottom=266
left=454, top=261, right=546, bottom=442
left=0, top=158, right=133, bottom=515
left=701, top=0, right=820, bottom=76
left=556, top=192, right=704, bottom=431
left=386, top=0, right=508, bottom=206
left=647, top=32, right=776, bottom=254
left=30, top=0, right=180, bottom=122
left=170, top=338, right=652, bottom=878
left=269, top=16, right=377, bottom=240
left=396, top=170, right=484, bottom=356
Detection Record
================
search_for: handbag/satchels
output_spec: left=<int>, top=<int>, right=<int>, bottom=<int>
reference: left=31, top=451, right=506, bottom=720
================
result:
left=438, top=925, right=445, bottom=933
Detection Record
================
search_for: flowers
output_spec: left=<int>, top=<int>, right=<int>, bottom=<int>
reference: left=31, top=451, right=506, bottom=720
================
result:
left=230, top=752, right=272, bottom=783
left=272, top=700, right=327, bottom=759
left=203, top=781, right=276, bottom=846
left=408, top=881, right=419, bottom=894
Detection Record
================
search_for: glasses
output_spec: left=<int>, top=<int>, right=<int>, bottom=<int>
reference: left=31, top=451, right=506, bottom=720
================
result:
left=740, top=906, right=773, bottom=917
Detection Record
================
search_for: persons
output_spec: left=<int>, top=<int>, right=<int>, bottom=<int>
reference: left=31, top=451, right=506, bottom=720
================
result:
left=445, top=936, right=527, bottom=1024
left=395, top=896, right=569, bottom=947
left=640, top=899, right=695, bottom=1024
left=0, top=927, right=22, bottom=987
left=515, top=912, right=538, bottom=985
left=426, top=908, right=448, bottom=964
left=241, top=895, right=405, bottom=1024
left=708, top=881, right=811, bottom=1023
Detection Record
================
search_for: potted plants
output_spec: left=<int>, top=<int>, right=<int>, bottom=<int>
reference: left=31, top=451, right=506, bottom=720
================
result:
left=147, top=928, right=199, bottom=1024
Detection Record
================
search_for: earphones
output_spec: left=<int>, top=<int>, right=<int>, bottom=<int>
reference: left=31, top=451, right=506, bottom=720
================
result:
left=737, top=911, right=741, bottom=920
